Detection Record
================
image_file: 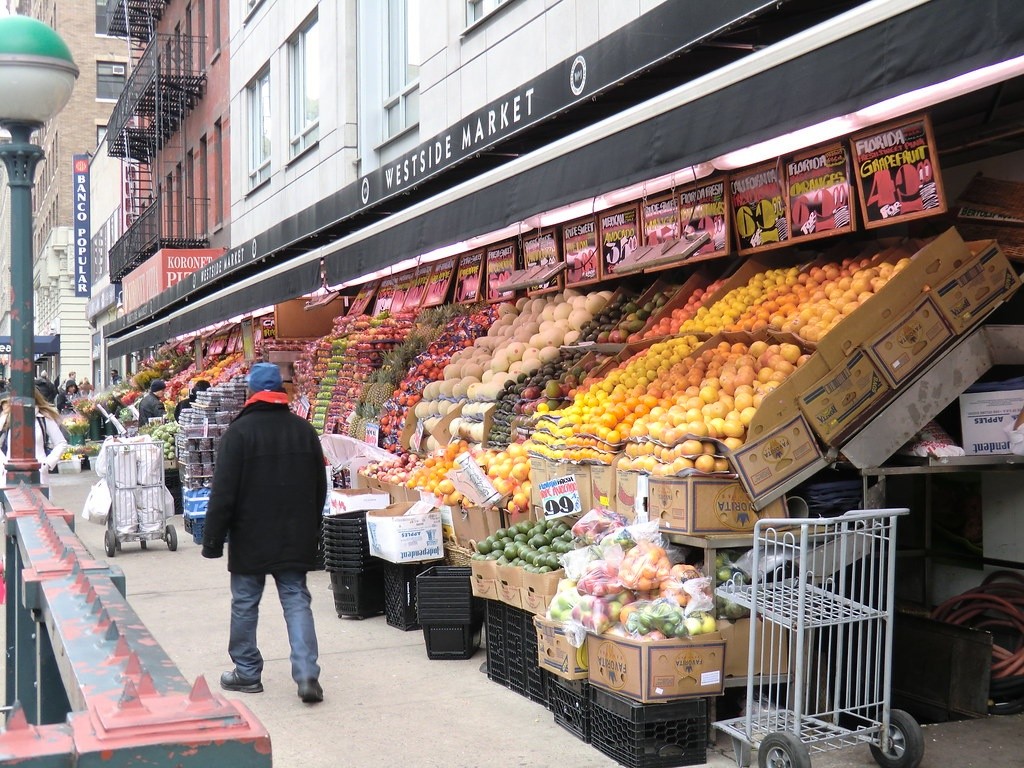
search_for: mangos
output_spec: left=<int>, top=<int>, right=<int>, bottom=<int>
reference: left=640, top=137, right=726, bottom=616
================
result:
left=150, top=425, right=178, bottom=459
left=518, top=289, right=673, bottom=443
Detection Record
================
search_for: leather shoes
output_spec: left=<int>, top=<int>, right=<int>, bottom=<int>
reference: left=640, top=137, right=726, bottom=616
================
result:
left=220, top=668, right=263, bottom=693
left=298, top=679, right=323, bottom=702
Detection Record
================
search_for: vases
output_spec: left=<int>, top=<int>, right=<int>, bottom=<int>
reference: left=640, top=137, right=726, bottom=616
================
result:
left=69, top=434, right=84, bottom=445
left=104, top=418, right=116, bottom=435
left=89, top=415, right=101, bottom=441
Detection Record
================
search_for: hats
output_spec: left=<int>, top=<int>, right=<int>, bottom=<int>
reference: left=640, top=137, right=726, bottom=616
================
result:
left=151, top=381, right=166, bottom=392
left=245, top=363, right=282, bottom=392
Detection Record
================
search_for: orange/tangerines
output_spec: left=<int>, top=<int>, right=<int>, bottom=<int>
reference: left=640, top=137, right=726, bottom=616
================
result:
left=619, top=539, right=757, bottom=644
left=180, top=355, right=236, bottom=398
left=404, top=440, right=533, bottom=513
left=378, top=300, right=516, bottom=453
left=672, top=255, right=911, bottom=342
left=533, top=316, right=806, bottom=470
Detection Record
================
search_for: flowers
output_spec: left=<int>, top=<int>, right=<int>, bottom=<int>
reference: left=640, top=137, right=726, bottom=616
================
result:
left=60, top=445, right=101, bottom=461
left=64, top=343, right=194, bottom=436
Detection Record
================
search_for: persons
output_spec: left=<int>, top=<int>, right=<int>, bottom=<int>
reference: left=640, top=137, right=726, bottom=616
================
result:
left=57, top=372, right=76, bottom=395
left=138, top=379, right=166, bottom=430
left=80, top=377, right=90, bottom=385
left=111, top=369, right=122, bottom=385
left=174, top=380, right=211, bottom=423
left=201, top=362, right=327, bottom=702
left=34, top=371, right=56, bottom=402
left=57, top=380, right=78, bottom=414
left=0, top=378, right=67, bottom=486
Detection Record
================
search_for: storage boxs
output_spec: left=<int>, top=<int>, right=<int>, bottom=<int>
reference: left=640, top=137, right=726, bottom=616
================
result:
left=183, top=507, right=230, bottom=544
left=321, top=226, right=1023, bottom=768
left=56, top=458, right=82, bottom=473
left=88, top=456, right=99, bottom=472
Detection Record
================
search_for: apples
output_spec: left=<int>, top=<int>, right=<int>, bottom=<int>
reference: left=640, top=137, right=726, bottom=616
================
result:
left=289, top=307, right=418, bottom=435
left=358, top=454, right=425, bottom=486
left=546, top=506, right=640, bottom=634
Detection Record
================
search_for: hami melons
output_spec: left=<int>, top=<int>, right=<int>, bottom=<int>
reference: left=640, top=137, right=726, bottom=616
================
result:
left=408, top=288, right=613, bottom=453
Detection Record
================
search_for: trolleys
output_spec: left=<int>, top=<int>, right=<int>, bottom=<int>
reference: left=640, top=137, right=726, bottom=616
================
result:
left=710, top=508, right=925, bottom=768
left=105, top=440, right=177, bottom=558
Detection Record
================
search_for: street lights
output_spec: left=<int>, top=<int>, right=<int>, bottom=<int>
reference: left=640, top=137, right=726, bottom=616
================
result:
left=0, top=16, right=81, bottom=725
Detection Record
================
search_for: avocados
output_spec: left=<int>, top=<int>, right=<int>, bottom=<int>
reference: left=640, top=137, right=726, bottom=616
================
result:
left=488, top=285, right=645, bottom=449
left=473, top=518, right=581, bottom=575
